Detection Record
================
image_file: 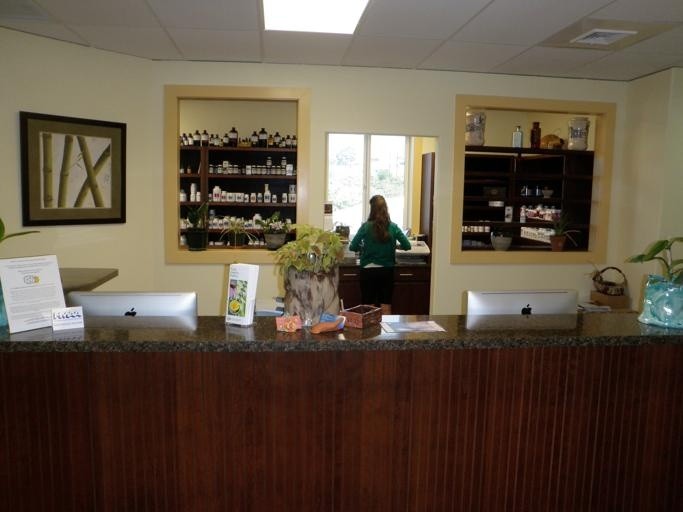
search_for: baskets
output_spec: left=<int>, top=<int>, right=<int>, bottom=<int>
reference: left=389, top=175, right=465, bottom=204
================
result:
left=592, top=267, right=627, bottom=295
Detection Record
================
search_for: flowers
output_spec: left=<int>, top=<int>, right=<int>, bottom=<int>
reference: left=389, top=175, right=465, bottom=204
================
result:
left=254, top=211, right=290, bottom=233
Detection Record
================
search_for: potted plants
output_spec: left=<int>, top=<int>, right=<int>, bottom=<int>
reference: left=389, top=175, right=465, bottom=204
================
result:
left=268, top=223, right=353, bottom=328
left=184, top=200, right=210, bottom=251
left=548, top=208, right=583, bottom=251
left=623, top=236, right=683, bottom=314
left=215, top=214, right=260, bottom=249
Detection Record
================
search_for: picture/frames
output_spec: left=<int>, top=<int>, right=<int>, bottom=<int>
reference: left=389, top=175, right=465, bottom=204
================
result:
left=19, top=110, right=127, bottom=227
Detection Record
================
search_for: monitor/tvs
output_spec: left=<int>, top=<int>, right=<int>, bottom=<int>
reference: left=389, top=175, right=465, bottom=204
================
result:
left=68, top=291, right=198, bottom=317
left=467, top=291, right=578, bottom=315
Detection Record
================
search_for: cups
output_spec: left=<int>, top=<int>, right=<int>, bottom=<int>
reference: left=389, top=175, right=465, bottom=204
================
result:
left=288, top=184, right=297, bottom=203
left=461, top=226, right=491, bottom=233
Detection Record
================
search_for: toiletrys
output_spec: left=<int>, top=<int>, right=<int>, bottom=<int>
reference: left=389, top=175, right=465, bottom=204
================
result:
left=513, top=125, right=523, bottom=148
left=193, top=127, right=268, bottom=148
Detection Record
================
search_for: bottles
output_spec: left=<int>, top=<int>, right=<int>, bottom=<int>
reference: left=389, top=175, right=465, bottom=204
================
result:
left=536, top=187, right=541, bottom=196
left=179, top=181, right=288, bottom=203
left=512, top=126, right=523, bottom=147
left=180, top=156, right=288, bottom=175
left=520, top=203, right=559, bottom=224
left=322, top=200, right=334, bottom=232
left=206, top=209, right=291, bottom=230
left=180, top=235, right=264, bottom=246
left=180, top=127, right=297, bottom=149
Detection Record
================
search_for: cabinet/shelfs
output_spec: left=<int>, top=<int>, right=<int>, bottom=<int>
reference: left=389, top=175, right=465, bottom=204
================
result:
left=180, top=145, right=298, bottom=250
left=392, top=265, right=431, bottom=315
left=338, top=266, right=363, bottom=309
left=463, top=146, right=595, bottom=251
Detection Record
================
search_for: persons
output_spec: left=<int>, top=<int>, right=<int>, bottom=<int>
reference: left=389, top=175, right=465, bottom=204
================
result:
left=349, top=193, right=412, bottom=314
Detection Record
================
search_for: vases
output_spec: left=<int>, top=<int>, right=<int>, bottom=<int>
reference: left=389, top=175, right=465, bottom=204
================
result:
left=490, top=235, right=513, bottom=252
left=567, top=116, right=591, bottom=151
left=466, top=112, right=486, bottom=145
left=264, top=234, right=287, bottom=249
